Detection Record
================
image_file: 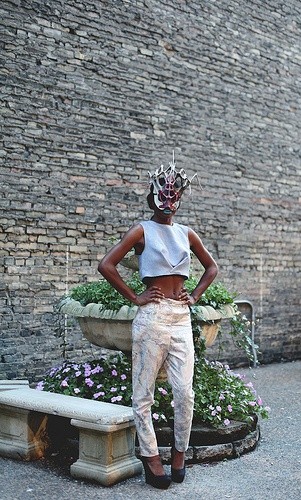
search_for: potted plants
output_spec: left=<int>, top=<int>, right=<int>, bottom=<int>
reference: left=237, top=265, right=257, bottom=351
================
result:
left=57, top=238, right=260, bottom=383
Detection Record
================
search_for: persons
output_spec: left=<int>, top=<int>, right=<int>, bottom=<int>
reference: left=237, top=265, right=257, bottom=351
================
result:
left=97, top=150, right=219, bottom=489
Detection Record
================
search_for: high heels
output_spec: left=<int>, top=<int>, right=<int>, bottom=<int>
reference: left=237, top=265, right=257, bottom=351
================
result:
left=141, top=458, right=172, bottom=489
left=170, top=446, right=186, bottom=483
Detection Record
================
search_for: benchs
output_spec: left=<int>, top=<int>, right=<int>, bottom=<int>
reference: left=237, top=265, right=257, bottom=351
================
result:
left=0, top=379, right=143, bottom=487
